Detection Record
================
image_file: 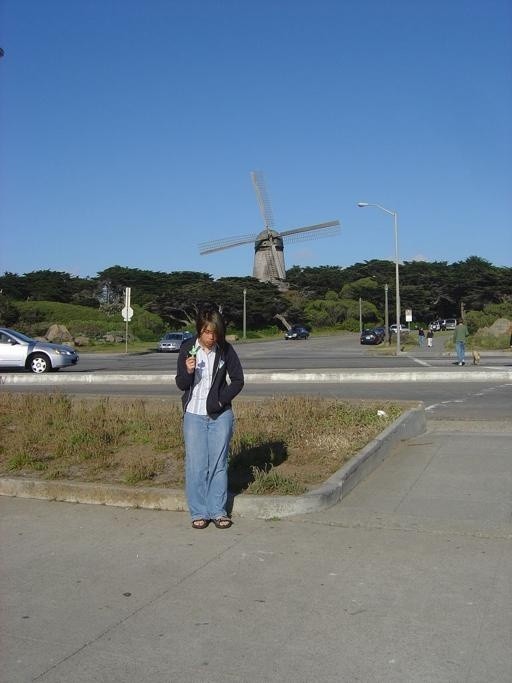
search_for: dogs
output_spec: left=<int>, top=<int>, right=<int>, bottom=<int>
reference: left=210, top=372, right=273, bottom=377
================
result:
left=472, top=350, right=481, bottom=366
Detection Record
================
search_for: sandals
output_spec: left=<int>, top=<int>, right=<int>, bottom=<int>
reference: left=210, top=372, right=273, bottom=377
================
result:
left=210, top=515, right=232, bottom=529
left=191, top=519, right=210, bottom=529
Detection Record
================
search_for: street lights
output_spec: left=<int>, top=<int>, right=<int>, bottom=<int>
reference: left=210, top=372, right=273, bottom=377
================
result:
left=383, top=281, right=390, bottom=342
left=357, top=200, right=402, bottom=354
left=242, top=287, right=249, bottom=340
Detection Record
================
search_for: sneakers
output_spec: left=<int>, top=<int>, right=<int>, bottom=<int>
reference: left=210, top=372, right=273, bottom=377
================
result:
left=462, top=359, right=465, bottom=362
left=458, top=361, right=462, bottom=365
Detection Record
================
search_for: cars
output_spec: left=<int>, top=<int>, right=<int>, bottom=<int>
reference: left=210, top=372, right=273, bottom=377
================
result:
left=283, top=324, right=311, bottom=340
left=358, top=322, right=411, bottom=345
left=156, top=330, right=194, bottom=352
left=0, top=328, right=81, bottom=374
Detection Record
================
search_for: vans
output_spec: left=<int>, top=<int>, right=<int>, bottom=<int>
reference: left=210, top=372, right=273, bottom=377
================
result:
left=427, top=318, right=459, bottom=332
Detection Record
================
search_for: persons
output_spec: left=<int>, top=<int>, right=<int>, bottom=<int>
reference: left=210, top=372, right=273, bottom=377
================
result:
left=419, top=327, right=424, bottom=348
left=175, top=306, right=244, bottom=529
left=509, top=332, right=512, bottom=349
left=453, top=316, right=469, bottom=365
left=427, top=329, right=433, bottom=347
